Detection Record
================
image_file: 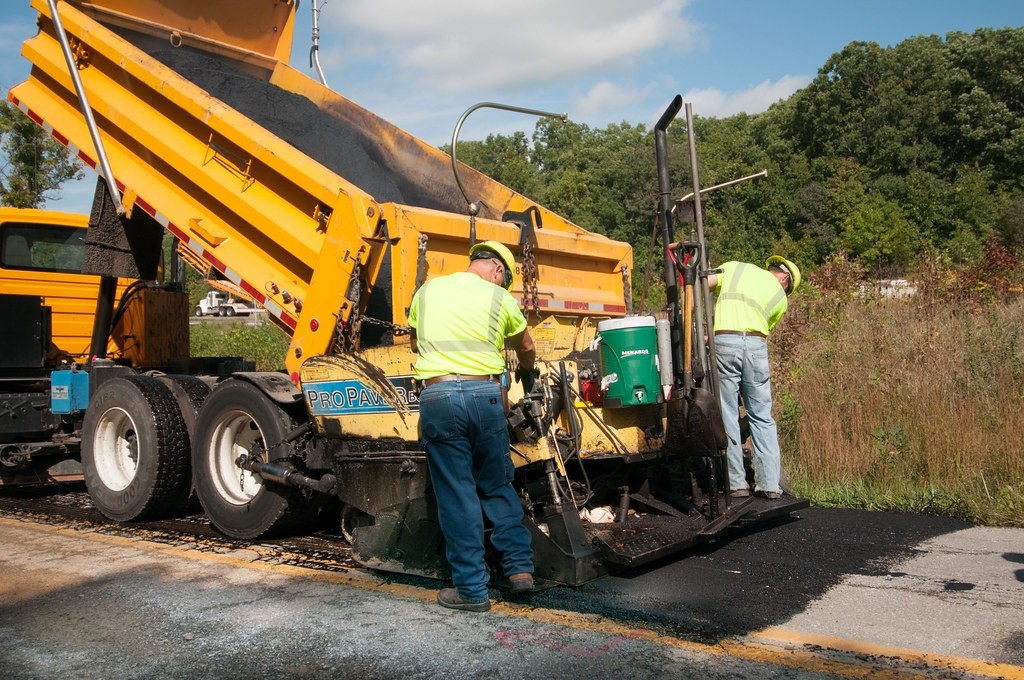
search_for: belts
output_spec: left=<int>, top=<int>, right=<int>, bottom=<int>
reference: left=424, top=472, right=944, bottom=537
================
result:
left=715, top=330, right=766, bottom=337
left=421, top=374, right=499, bottom=388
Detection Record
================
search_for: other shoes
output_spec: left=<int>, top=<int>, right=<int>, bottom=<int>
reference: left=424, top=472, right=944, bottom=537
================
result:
left=763, top=491, right=779, bottom=498
left=730, top=490, right=750, bottom=498
left=508, top=571, right=536, bottom=592
left=437, top=588, right=492, bottom=612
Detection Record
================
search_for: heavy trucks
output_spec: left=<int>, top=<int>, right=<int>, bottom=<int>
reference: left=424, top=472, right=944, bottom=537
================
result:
left=0, top=0, right=813, bottom=590
left=195, top=292, right=260, bottom=317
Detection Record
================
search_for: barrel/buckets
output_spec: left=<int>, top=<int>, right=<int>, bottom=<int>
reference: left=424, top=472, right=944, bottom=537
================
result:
left=597, top=317, right=661, bottom=405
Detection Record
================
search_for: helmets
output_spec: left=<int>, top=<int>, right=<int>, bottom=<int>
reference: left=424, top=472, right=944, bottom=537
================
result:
left=765, top=255, right=801, bottom=298
left=469, top=241, right=515, bottom=293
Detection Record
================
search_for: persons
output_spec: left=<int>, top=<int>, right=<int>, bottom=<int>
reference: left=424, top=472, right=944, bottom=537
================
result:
left=707, top=254, right=802, bottom=499
left=409, top=240, right=536, bottom=613
